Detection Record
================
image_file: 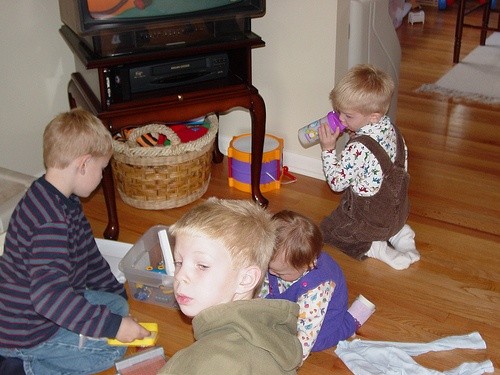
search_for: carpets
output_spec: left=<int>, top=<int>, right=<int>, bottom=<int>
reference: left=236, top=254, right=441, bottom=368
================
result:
left=413, top=30, right=500, bottom=106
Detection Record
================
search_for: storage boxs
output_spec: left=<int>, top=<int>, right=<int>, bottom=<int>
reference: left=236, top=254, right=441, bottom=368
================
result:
left=117, top=225, right=179, bottom=312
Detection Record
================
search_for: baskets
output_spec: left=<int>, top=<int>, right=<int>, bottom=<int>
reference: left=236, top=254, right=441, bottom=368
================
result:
left=112, top=111, right=219, bottom=210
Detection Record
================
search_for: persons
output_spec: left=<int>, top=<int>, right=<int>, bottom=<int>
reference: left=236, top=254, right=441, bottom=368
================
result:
left=156, top=197, right=303, bottom=375
left=0, top=106, right=152, bottom=375
left=319, top=62, right=421, bottom=271
left=257, top=210, right=376, bottom=371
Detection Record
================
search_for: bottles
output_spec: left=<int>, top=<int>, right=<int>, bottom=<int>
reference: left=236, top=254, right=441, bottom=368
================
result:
left=296, top=110, right=351, bottom=149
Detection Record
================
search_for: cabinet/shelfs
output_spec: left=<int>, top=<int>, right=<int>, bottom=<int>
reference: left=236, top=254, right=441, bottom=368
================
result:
left=56, top=0, right=269, bottom=239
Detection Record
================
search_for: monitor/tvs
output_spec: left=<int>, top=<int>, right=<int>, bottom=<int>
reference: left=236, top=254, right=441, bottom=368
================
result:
left=58, top=0, right=267, bottom=58
